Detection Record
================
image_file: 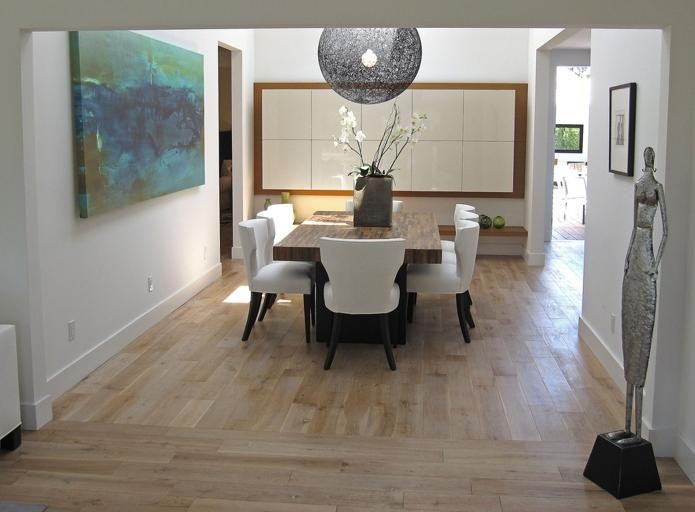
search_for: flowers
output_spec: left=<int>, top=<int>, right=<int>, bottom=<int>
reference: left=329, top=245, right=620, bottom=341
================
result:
left=329, top=97, right=428, bottom=191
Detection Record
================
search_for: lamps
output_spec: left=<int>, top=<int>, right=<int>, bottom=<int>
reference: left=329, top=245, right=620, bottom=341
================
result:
left=318, top=28, right=422, bottom=105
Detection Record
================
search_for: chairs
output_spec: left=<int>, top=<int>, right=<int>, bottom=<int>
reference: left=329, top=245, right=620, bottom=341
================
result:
left=237, top=218, right=316, bottom=341
left=256, top=210, right=320, bottom=310
left=346, top=199, right=404, bottom=213
left=267, top=203, right=300, bottom=235
left=441, top=211, right=479, bottom=306
left=436, top=204, right=476, bottom=253
left=319, top=237, right=406, bottom=371
left=406, top=219, right=479, bottom=343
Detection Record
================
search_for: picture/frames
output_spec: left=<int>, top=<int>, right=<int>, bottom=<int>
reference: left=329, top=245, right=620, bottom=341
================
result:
left=70, top=31, right=206, bottom=219
left=608, top=82, right=637, bottom=177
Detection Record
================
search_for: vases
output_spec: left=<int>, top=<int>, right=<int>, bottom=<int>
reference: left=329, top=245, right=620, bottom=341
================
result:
left=478, top=214, right=485, bottom=226
left=264, top=198, right=272, bottom=210
left=277, top=192, right=297, bottom=223
left=354, top=176, right=393, bottom=228
left=493, top=216, right=505, bottom=228
left=480, top=216, right=492, bottom=228
